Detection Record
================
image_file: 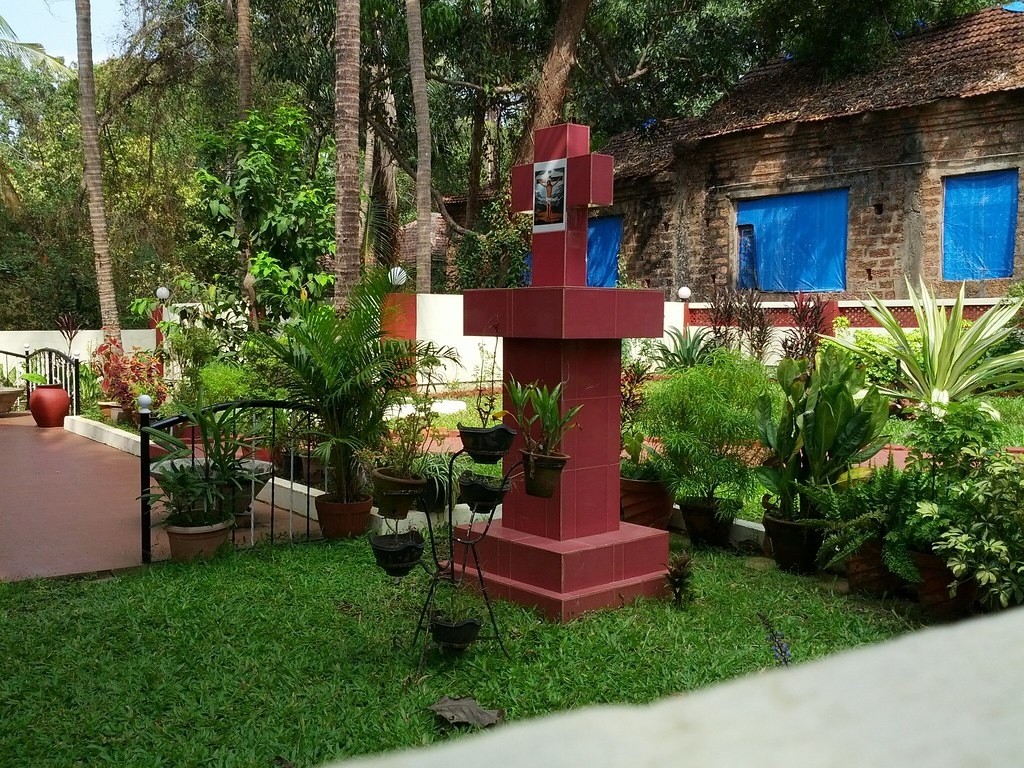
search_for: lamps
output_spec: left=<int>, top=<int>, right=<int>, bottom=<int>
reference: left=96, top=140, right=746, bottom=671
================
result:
left=387, top=266, right=407, bottom=293
left=156, top=287, right=169, bottom=304
left=678, top=287, right=691, bottom=303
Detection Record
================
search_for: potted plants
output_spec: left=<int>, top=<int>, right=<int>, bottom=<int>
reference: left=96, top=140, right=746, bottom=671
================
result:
left=0, top=260, right=1024, bottom=652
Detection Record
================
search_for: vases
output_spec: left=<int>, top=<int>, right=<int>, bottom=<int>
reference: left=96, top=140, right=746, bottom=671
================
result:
left=29, top=384, right=70, bottom=428
